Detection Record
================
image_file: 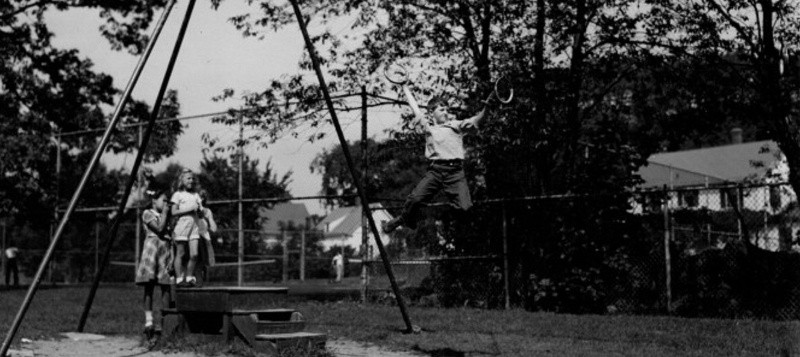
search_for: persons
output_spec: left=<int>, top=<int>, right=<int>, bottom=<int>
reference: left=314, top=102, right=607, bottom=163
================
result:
left=2, top=247, right=20, bottom=287
left=133, top=168, right=216, bottom=341
left=381, top=83, right=495, bottom=234
left=331, top=250, right=347, bottom=284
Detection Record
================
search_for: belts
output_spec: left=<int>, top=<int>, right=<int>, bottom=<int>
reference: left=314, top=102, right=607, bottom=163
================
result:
left=434, top=160, right=462, bottom=165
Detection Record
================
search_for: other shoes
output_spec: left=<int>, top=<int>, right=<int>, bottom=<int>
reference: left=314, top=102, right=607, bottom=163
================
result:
left=144, top=320, right=155, bottom=331
left=175, top=276, right=184, bottom=286
left=186, top=276, right=196, bottom=287
left=383, top=216, right=402, bottom=233
